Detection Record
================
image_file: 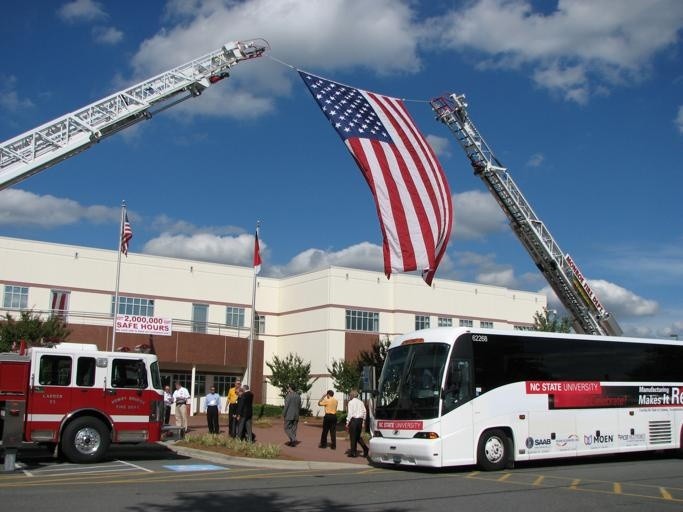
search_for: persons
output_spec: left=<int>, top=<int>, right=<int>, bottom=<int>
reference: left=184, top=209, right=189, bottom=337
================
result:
left=280, top=383, right=301, bottom=447
left=200, top=386, right=221, bottom=435
left=223, top=377, right=256, bottom=444
left=343, top=390, right=369, bottom=458
left=316, top=389, right=338, bottom=450
left=169, top=381, right=191, bottom=435
left=163, top=384, right=173, bottom=425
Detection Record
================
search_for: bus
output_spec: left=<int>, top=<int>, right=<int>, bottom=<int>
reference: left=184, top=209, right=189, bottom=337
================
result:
left=366, top=326, right=683, bottom=470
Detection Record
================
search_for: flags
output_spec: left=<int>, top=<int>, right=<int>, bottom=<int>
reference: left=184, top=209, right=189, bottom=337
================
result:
left=251, top=226, right=263, bottom=276
left=118, top=208, right=135, bottom=258
left=295, top=66, right=454, bottom=288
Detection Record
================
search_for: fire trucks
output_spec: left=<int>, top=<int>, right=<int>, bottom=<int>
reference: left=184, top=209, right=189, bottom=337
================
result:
left=0, top=339, right=166, bottom=464
